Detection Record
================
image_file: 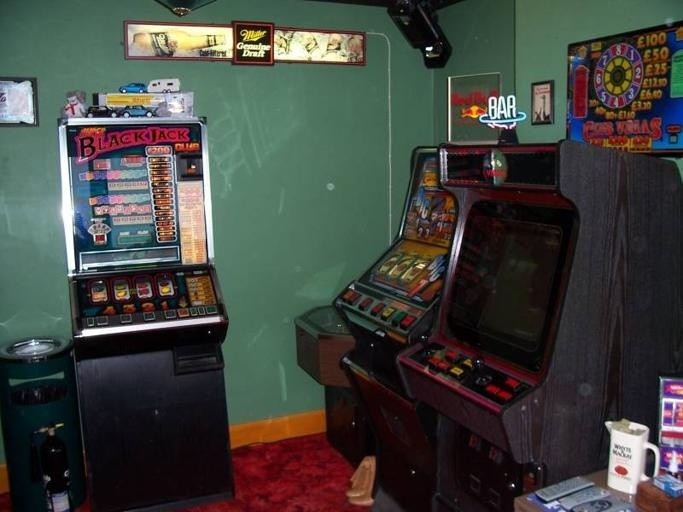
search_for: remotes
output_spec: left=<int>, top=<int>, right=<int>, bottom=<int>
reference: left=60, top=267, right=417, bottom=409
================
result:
left=558, top=486, right=609, bottom=506
left=535, top=476, right=594, bottom=502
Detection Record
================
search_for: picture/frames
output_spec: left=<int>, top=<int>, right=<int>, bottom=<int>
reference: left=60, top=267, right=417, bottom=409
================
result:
left=532, top=80, right=556, bottom=125
left=124, top=20, right=366, bottom=66
left=0, top=77, right=38, bottom=127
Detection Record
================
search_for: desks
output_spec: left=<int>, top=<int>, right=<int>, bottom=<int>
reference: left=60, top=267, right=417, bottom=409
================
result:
left=515, top=467, right=683, bottom=512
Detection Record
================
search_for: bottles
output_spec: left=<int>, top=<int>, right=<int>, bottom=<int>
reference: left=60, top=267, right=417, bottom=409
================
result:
left=39, top=426, right=77, bottom=512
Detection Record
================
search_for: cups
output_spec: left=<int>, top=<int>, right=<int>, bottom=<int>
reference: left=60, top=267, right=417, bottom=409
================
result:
left=604, top=419, right=662, bottom=497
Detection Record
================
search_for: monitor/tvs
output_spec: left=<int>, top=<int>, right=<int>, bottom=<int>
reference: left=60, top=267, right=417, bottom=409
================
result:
left=448, top=203, right=567, bottom=366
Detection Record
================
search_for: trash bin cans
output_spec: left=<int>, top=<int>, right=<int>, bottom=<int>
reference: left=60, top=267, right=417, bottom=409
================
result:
left=0, top=334, right=86, bottom=512
